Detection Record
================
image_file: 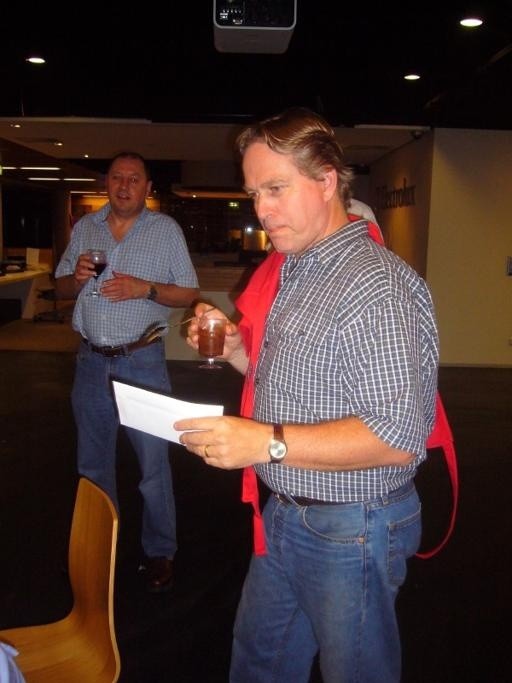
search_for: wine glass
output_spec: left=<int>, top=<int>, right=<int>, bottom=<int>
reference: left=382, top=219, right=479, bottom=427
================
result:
left=86, top=249, right=107, bottom=297
left=198, top=319, right=227, bottom=369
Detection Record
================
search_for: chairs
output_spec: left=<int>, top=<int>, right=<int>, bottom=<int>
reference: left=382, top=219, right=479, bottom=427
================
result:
left=1, top=476, right=123, bottom=682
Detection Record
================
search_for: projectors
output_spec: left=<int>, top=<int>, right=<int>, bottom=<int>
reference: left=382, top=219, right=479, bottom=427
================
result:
left=211, top=0, right=298, bottom=55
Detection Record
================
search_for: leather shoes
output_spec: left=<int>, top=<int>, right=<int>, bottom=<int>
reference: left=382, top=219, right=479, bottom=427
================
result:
left=135, top=555, right=171, bottom=610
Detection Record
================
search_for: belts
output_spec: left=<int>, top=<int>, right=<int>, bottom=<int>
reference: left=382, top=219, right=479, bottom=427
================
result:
left=273, top=492, right=322, bottom=506
left=81, top=336, right=159, bottom=358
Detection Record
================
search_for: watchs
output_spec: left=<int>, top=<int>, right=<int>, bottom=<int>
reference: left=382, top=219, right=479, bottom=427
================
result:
left=144, top=280, right=160, bottom=301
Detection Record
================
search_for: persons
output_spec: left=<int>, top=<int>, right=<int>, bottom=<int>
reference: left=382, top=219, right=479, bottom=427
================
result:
left=56, top=151, right=201, bottom=587
left=175, top=109, right=440, bottom=682
